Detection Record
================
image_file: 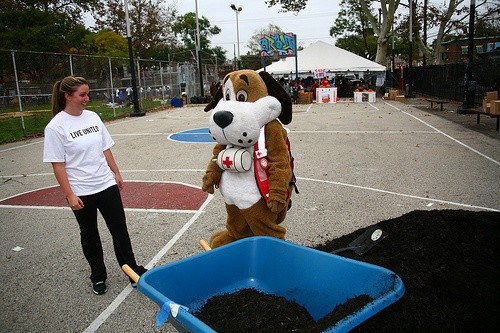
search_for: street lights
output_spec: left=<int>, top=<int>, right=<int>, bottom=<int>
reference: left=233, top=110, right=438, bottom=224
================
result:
left=230, top=4, right=242, bottom=71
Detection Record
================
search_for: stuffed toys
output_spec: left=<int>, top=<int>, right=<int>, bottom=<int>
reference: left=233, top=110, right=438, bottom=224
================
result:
left=201, top=70, right=296, bottom=247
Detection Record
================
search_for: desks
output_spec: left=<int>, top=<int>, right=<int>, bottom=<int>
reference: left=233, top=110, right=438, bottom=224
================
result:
left=427, top=98, right=449, bottom=111
left=316, top=87, right=337, bottom=103
left=354, top=92, right=376, bottom=102
left=472, top=107, right=500, bottom=131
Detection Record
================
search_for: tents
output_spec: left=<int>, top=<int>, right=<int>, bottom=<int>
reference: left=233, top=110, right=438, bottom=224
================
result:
left=255, top=40, right=387, bottom=97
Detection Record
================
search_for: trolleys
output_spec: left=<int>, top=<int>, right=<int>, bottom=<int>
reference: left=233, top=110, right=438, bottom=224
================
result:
left=121, top=236, right=406, bottom=333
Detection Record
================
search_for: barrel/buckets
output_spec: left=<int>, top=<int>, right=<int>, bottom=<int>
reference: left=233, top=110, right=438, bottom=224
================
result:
left=212, top=149, right=252, bottom=174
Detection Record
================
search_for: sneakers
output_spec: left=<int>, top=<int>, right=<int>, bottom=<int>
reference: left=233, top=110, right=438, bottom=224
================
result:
left=129, top=270, right=149, bottom=287
left=92, top=279, right=107, bottom=294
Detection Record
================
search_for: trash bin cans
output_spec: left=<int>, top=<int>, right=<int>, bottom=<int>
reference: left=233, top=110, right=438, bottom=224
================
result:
left=463, top=90, right=476, bottom=108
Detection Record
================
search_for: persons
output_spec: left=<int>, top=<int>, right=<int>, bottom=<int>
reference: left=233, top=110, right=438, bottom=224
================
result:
left=275, top=76, right=335, bottom=96
left=116, top=85, right=172, bottom=101
left=43, top=77, right=148, bottom=293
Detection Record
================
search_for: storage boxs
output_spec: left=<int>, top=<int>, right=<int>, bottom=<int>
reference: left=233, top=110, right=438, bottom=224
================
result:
left=483, top=92, right=500, bottom=115
left=384, top=90, right=405, bottom=101
